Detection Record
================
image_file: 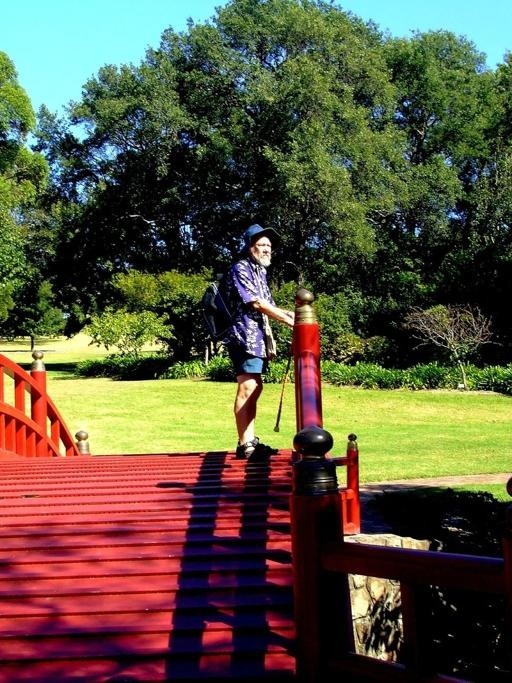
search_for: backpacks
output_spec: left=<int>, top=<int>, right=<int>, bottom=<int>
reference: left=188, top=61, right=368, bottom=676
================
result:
left=199, top=279, right=235, bottom=340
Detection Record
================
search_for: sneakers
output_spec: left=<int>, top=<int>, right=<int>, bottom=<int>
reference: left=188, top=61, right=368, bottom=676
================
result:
left=235, top=440, right=270, bottom=459
left=253, top=436, right=278, bottom=454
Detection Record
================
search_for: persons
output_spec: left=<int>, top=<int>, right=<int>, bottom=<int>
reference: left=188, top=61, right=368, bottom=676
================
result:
left=219, top=224, right=295, bottom=459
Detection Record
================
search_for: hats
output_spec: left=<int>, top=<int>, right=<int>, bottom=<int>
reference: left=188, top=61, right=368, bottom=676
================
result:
left=236, top=225, right=282, bottom=256
